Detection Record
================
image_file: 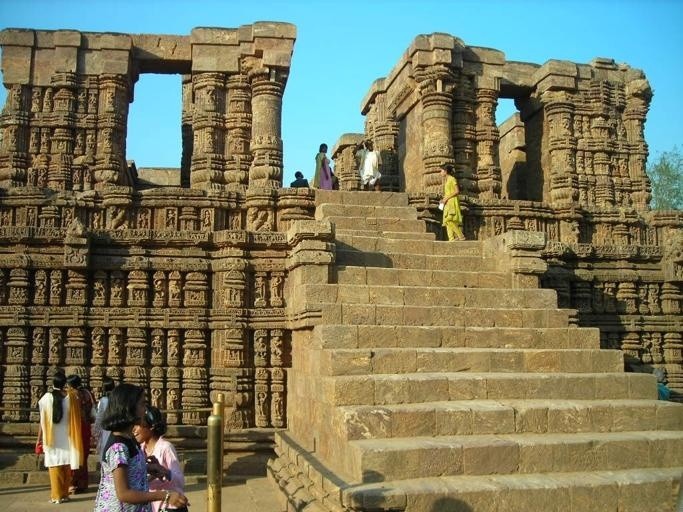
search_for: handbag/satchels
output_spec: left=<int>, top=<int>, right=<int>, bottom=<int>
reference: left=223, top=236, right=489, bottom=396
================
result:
left=156, top=491, right=189, bottom=512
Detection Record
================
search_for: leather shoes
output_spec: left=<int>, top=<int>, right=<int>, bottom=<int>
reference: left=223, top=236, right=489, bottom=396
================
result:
left=48, top=497, right=67, bottom=505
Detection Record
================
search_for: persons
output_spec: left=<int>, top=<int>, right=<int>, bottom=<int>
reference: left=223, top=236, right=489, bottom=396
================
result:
left=436, top=161, right=466, bottom=241
left=358, top=138, right=383, bottom=190
left=312, top=142, right=334, bottom=190
left=290, top=171, right=310, bottom=187
left=93, top=377, right=116, bottom=463
left=63, top=374, right=95, bottom=495
left=35, top=371, right=85, bottom=505
left=652, top=366, right=671, bottom=400
left=132, top=406, right=187, bottom=512
left=93, top=383, right=190, bottom=511
